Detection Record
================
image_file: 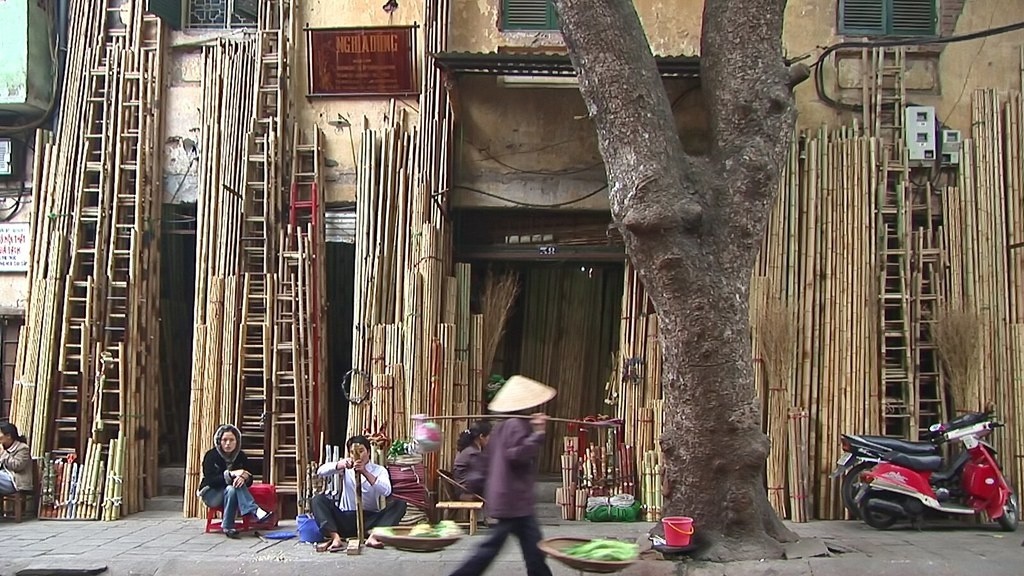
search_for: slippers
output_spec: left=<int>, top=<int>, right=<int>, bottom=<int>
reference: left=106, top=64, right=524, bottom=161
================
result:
left=224, top=530, right=241, bottom=539
left=253, top=505, right=273, bottom=524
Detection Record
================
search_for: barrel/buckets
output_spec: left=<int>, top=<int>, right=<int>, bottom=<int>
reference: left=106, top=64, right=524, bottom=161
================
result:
left=661, top=516, right=694, bottom=547
left=296, top=515, right=324, bottom=543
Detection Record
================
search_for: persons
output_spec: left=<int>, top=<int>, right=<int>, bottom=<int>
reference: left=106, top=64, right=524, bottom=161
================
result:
left=0, top=422, right=33, bottom=495
left=198, top=424, right=273, bottom=539
left=309, top=436, right=407, bottom=551
left=447, top=375, right=552, bottom=576
left=454, top=421, right=499, bottom=529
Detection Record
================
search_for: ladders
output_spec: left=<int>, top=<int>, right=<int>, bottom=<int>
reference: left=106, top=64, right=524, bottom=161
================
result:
left=234, top=29, right=320, bottom=515
left=872, top=46, right=949, bottom=463
left=50, top=1, right=163, bottom=514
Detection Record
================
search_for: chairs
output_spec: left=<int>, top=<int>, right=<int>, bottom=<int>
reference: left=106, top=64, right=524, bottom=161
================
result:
left=436, top=469, right=489, bottom=528
left=0, top=457, right=38, bottom=523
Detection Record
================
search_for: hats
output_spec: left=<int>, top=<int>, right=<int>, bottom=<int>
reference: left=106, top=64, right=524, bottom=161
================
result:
left=487, top=376, right=557, bottom=412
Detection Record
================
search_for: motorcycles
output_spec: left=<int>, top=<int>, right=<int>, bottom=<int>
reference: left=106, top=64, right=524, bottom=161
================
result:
left=825, top=399, right=1021, bottom=531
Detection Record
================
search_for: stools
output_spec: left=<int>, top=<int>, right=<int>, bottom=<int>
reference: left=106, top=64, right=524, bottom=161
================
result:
left=437, top=501, right=484, bottom=535
left=206, top=502, right=250, bottom=534
